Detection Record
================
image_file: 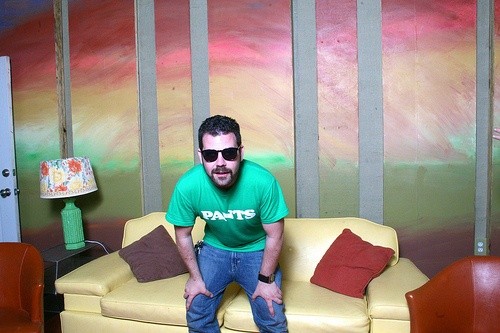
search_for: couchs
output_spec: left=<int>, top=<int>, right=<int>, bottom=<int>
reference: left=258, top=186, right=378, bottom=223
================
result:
left=55, top=213, right=429, bottom=333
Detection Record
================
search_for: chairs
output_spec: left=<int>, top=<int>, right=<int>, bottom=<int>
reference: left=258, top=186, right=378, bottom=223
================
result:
left=0, top=242, right=45, bottom=333
left=405, top=256, right=500, bottom=333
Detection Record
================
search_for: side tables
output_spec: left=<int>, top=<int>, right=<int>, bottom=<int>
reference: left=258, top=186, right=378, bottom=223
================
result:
left=40, top=240, right=104, bottom=315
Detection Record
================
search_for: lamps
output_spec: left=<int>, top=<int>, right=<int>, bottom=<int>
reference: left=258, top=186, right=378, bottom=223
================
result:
left=39, top=156, right=98, bottom=250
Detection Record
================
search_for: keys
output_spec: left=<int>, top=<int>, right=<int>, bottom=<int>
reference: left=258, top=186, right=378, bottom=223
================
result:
left=194, top=240, right=205, bottom=256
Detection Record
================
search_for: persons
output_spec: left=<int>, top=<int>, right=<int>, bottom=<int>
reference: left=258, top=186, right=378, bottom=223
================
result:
left=165, top=115, right=289, bottom=333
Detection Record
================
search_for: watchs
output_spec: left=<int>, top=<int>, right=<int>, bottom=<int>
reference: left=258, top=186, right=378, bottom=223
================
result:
left=258, top=273, right=275, bottom=283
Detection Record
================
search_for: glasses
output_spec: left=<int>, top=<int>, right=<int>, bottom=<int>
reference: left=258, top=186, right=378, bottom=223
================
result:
left=200, top=146, right=241, bottom=163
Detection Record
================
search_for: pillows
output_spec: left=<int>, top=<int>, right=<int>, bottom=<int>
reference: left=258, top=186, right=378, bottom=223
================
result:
left=310, top=228, right=395, bottom=298
left=119, top=225, right=189, bottom=283
